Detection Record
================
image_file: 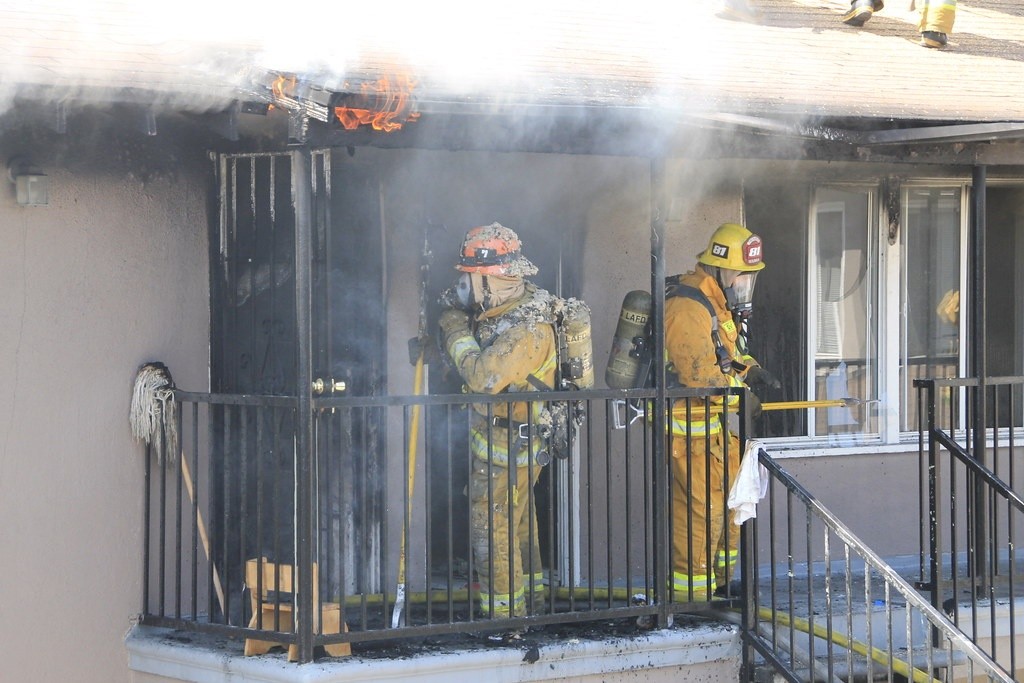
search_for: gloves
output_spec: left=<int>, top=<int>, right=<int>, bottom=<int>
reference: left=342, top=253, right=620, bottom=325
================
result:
left=408, top=336, right=437, bottom=366
left=438, top=310, right=470, bottom=341
left=736, top=390, right=762, bottom=422
left=747, top=365, right=782, bottom=393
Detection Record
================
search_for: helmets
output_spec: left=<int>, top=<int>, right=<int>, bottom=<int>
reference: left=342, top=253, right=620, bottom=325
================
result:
left=696, top=224, right=766, bottom=272
left=453, top=224, right=537, bottom=276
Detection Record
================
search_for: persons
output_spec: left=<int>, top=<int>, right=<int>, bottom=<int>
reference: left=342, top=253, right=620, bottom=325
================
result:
left=637, top=223, right=781, bottom=602
left=436, top=222, right=556, bottom=637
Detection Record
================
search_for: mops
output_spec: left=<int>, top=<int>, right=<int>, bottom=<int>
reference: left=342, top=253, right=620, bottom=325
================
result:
left=128, top=361, right=236, bottom=640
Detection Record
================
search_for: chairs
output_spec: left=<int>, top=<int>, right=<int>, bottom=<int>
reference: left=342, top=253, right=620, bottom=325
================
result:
left=244, top=556, right=352, bottom=661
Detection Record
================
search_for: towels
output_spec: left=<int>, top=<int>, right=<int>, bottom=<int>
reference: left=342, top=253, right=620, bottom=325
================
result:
left=727, top=438, right=769, bottom=526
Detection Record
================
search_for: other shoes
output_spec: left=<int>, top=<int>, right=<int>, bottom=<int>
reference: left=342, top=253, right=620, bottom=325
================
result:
left=715, top=580, right=761, bottom=600
left=455, top=605, right=547, bottom=631
left=460, top=615, right=531, bottom=639
